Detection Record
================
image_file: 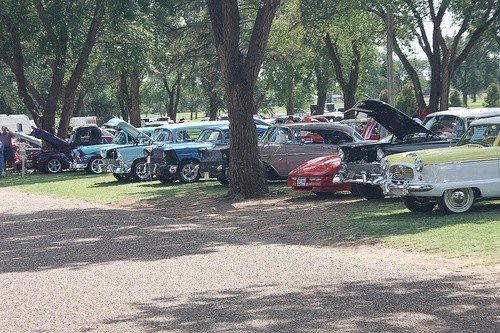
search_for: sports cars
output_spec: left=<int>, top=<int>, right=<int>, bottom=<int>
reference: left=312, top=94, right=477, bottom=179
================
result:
left=287, top=153, right=366, bottom=197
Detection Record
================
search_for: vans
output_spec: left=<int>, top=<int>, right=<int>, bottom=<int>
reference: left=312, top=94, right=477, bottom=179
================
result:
left=29, top=116, right=97, bottom=132
left=0, top=115, right=33, bottom=143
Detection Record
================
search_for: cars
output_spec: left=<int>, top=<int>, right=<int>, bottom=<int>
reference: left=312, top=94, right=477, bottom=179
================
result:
left=258, top=122, right=366, bottom=184
left=315, top=112, right=347, bottom=123
left=312, top=116, right=329, bottom=122
left=253, top=116, right=270, bottom=125
left=339, top=118, right=380, bottom=141
left=332, top=98, right=500, bottom=200
left=105, top=129, right=116, bottom=134
left=292, top=108, right=309, bottom=118
left=98, top=127, right=115, bottom=144
left=261, top=119, right=275, bottom=127
left=300, top=116, right=322, bottom=125
left=378, top=117, right=500, bottom=214
left=146, top=121, right=167, bottom=127
left=438, top=107, right=467, bottom=111
left=277, top=116, right=304, bottom=123
left=198, top=126, right=268, bottom=185
left=13, top=131, right=42, bottom=153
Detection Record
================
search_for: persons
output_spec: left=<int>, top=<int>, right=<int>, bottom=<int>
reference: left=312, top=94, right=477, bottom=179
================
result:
left=268, top=113, right=273, bottom=119
left=410, top=116, right=423, bottom=125
left=178, top=118, right=190, bottom=138
left=222, top=109, right=226, bottom=114
left=67, top=126, right=75, bottom=138
left=0, top=126, right=35, bottom=177
left=285, top=116, right=294, bottom=139
left=377, top=124, right=391, bottom=139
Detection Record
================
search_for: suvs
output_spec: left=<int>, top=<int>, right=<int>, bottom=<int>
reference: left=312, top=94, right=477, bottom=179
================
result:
left=69, top=126, right=154, bottom=174
left=99, top=119, right=230, bottom=183
left=143, top=124, right=230, bottom=183
left=31, top=125, right=104, bottom=174
